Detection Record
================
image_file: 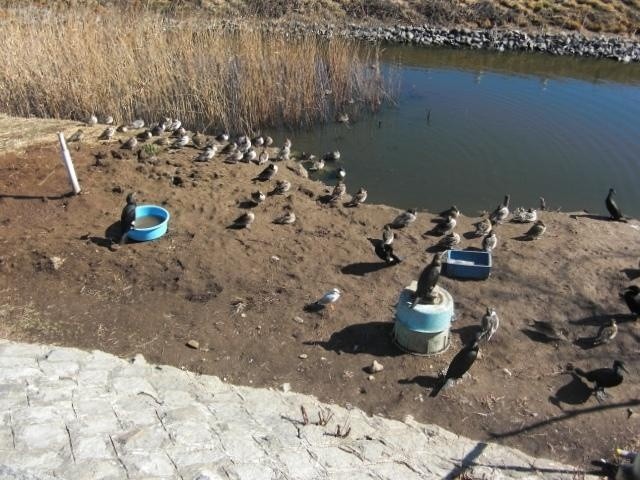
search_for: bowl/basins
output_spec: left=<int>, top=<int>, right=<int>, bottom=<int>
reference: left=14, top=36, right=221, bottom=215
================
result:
left=127, top=205, right=170, bottom=240
left=393, top=284, right=454, bottom=356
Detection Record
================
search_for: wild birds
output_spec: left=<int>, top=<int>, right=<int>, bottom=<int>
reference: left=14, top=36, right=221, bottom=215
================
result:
left=526, top=264, right=640, bottom=403
left=381, top=192, right=549, bottom=265
left=476, top=306, right=499, bottom=342
left=605, top=188, right=628, bottom=222
left=233, top=210, right=255, bottom=228
left=428, top=340, right=479, bottom=398
left=343, top=187, right=368, bottom=208
left=410, top=249, right=448, bottom=309
left=120, top=191, right=138, bottom=236
left=316, top=288, right=341, bottom=310
left=272, top=210, right=296, bottom=225
left=66, top=113, right=349, bottom=202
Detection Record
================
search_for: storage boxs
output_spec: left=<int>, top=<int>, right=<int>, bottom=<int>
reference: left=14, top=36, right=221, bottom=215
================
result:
left=447, top=250, right=492, bottom=279
